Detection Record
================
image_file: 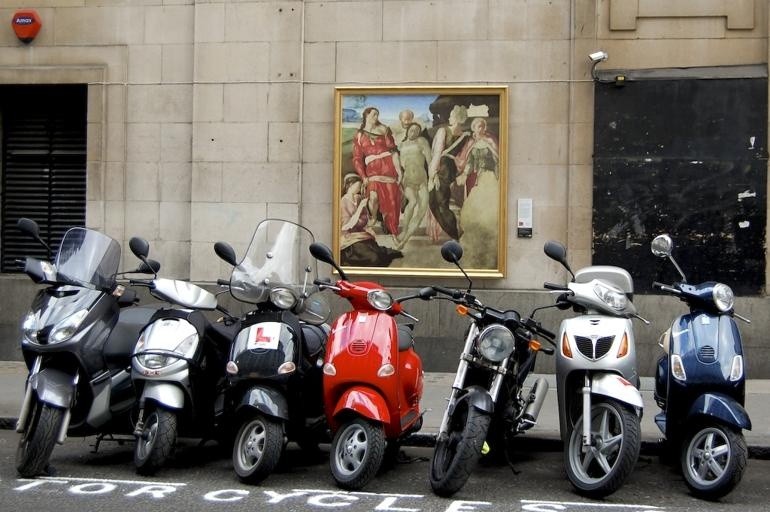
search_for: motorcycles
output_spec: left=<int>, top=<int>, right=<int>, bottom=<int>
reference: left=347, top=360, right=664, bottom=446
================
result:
left=428, top=241, right=558, bottom=499
left=544, top=240, right=650, bottom=497
left=129, top=237, right=244, bottom=477
left=307, top=241, right=439, bottom=492
left=15, top=219, right=161, bottom=479
left=652, top=232, right=751, bottom=499
left=214, top=219, right=332, bottom=486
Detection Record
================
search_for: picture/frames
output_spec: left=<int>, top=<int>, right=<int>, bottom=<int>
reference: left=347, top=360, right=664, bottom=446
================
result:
left=332, top=85, right=508, bottom=279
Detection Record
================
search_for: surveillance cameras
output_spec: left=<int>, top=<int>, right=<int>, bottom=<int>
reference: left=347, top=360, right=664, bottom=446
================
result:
left=588, top=52, right=604, bottom=62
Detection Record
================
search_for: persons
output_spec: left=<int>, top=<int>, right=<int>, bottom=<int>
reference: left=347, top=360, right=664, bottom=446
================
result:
left=341, top=104, right=500, bottom=268
